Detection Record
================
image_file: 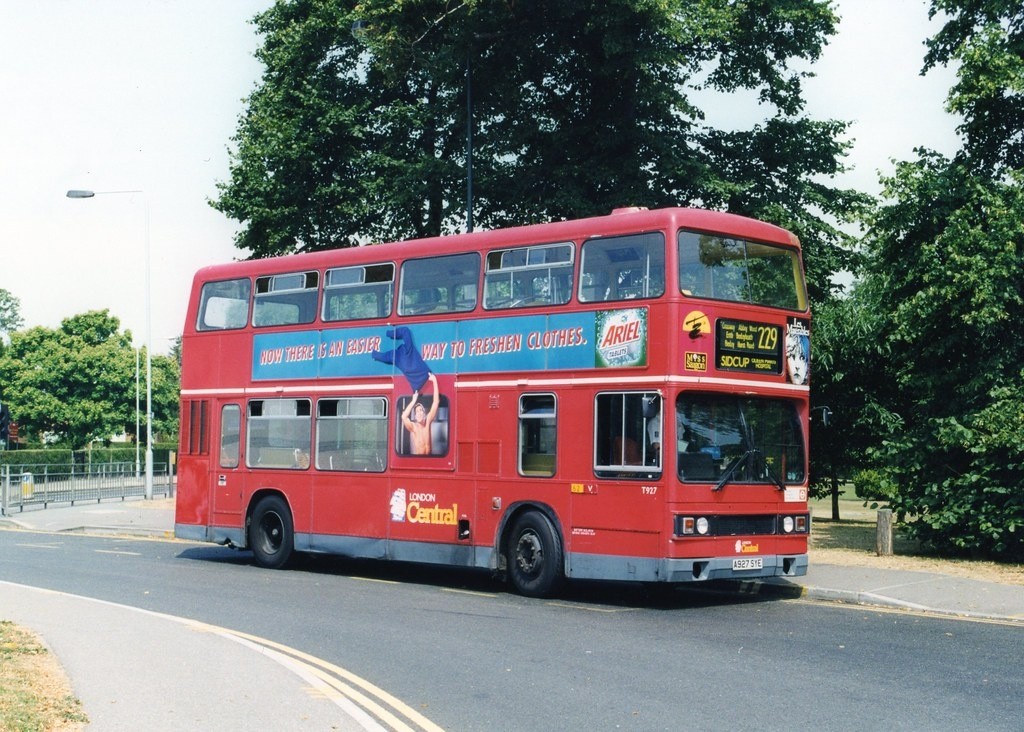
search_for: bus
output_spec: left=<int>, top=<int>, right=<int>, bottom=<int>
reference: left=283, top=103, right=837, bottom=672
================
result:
left=174, top=205, right=833, bottom=599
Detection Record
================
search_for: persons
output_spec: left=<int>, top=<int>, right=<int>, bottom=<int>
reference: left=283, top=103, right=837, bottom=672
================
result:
left=647, top=409, right=686, bottom=460
left=401, top=372, right=440, bottom=456
left=786, top=328, right=809, bottom=384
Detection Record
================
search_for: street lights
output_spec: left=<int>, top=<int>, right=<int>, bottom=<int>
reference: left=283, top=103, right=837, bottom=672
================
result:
left=64, top=190, right=154, bottom=499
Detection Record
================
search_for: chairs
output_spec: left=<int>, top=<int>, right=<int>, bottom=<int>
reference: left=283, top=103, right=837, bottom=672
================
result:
left=614, top=436, right=639, bottom=465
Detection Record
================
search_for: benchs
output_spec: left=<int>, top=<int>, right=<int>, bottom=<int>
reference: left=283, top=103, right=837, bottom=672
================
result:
left=258, top=446, right=386, bottom=471
